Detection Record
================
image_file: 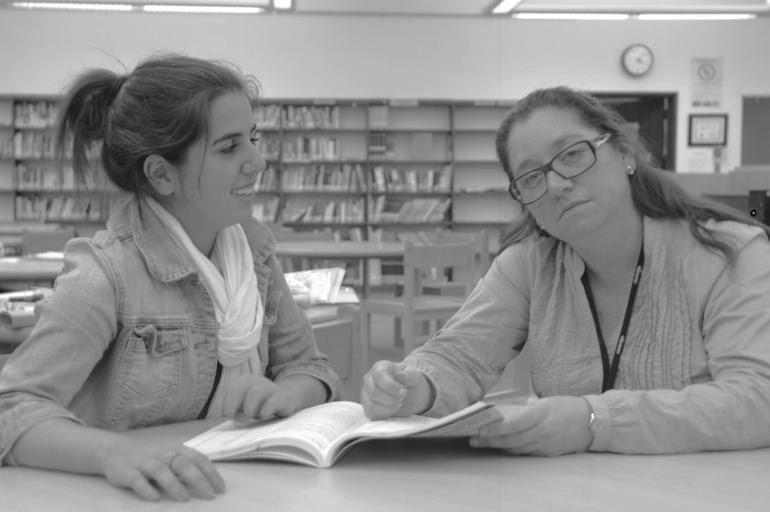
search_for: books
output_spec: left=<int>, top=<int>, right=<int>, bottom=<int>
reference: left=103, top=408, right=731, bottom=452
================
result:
left=176, top=398, right=508, bottom=471
left=284, top=228, right=365, bottom=282
left=375, top=194, right=452, bottom=220
left=0, top=96, right=122, bottom=333
left=256, top=132, right=341, bottom=159
left=258, top=164, right=367, bottom=191
left=368, top=105, right=390, bottom=161
left=254, top=198, right=365, bottom=222
left=253, top=104, right=340, bottom=129
left=378, top=227, right=450, bottom=279
left=370, top=165, right=450, bottom=190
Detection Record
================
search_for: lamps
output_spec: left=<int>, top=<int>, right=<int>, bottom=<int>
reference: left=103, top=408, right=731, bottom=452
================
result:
left=8, top=0, right=770, bottom=21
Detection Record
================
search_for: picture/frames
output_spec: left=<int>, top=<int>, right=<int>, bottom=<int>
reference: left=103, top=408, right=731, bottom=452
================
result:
left=688, top=114, right=728, bottom=148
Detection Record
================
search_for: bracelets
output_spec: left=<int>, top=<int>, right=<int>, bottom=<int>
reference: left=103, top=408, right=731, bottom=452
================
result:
left=586, top=409, right=596, bottom=438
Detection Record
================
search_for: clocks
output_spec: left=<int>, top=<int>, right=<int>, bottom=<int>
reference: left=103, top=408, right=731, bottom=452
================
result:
left=619, top=42, right=654, bottom=77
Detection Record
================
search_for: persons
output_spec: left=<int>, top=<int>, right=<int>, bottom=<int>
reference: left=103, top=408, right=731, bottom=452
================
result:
left=0, top=52, right=347, bottom=503
left=359, top=84, right=770, bottom=458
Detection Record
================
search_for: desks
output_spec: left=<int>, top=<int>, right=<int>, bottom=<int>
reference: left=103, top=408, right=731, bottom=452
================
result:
left=2, top=290, right=362, bottom=400
left=1, top=415, right=768, bottom=511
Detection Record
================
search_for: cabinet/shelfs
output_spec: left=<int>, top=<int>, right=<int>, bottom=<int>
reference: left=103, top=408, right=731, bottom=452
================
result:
left=0, top=93, right=531, bottom=290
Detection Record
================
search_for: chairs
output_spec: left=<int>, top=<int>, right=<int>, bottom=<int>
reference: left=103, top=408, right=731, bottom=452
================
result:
left=357, top=231, right=492, bottom=373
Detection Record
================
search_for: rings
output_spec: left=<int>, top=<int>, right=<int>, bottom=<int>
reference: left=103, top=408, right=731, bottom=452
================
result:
left=168, top=451, right=183, bottom=468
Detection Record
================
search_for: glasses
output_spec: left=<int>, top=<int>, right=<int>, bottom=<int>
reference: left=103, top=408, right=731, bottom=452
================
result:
left=508, top=129, right=615, bottom=207
